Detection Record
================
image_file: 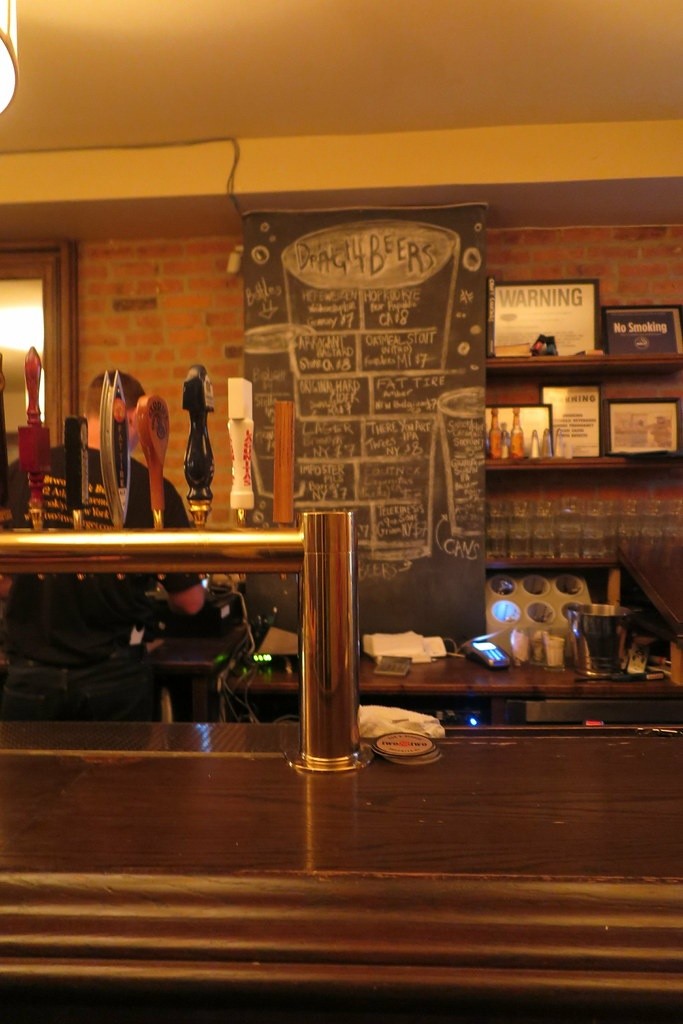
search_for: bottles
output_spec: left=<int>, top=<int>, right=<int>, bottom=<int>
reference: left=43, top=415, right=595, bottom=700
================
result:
left=500, top=423, right=510, bottom=441
left=541, top=428, right=552, bottom=458
left=510, top=407, right=523, bottom=459
left=489, top=408, right=501, bottom=460
left=530, top=430, right=539, bottom=459
left=502, top=432, right=508, bottom=458
left=555, top=428, right=563, bottom=458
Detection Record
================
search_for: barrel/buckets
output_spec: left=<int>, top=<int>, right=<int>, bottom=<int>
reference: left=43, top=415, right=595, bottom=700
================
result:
left=567, top=603, right=635, bottom=678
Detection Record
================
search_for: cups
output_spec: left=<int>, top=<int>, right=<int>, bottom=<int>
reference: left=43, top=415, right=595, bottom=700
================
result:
left=527, top=626, right=565, bottom=671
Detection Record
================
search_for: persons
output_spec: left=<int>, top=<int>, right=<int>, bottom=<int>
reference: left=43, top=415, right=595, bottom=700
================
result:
left=0, top=372, right=208, bottom=723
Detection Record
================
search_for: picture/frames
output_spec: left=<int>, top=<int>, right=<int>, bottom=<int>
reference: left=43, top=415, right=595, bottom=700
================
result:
left=602, top=396, right=683, bottom=455
left=486, top=404, right=553, bottom=461
